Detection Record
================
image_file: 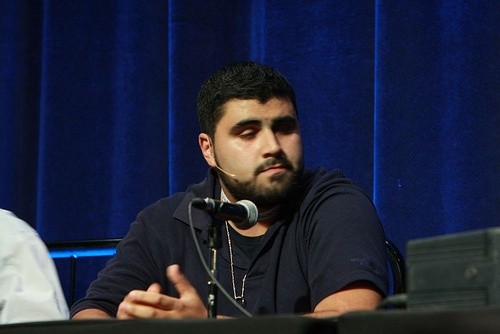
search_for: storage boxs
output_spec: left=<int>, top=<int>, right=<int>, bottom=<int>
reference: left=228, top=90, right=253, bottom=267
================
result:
left=406, top=228, right=500, bottom=314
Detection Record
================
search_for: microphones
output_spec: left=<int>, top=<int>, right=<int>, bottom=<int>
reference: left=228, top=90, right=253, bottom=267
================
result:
left=191, top=197, right=259, bottom=229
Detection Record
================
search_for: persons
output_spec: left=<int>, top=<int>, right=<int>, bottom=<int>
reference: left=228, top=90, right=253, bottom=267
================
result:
left=69, top=59, right=388, bottom=319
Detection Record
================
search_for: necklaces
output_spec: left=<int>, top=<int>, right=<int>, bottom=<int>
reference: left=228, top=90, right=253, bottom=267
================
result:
left=220, top=189, right=246, bottom=307
